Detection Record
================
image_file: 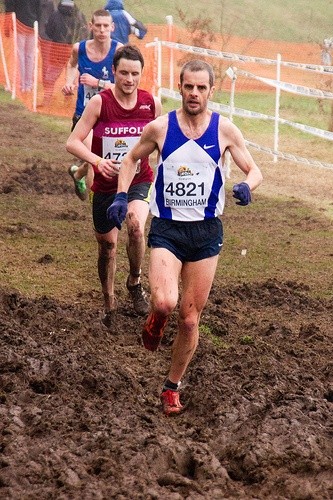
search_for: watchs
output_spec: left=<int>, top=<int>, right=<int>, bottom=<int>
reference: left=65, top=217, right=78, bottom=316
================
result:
left=98, top=78, right=105, bottom=91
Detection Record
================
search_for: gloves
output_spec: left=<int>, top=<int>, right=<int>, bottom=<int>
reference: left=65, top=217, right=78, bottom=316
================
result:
left=106, top=191, right=129, bottom=230
left=232, top=181, right=252, bottom=206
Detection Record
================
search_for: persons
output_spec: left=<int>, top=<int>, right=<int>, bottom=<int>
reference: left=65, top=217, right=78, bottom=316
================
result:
left=107, top=60, right=263, bottom=414
left=61, top=10, right=125, bottom=200
left=4, top=0, right=147, bottom=97
left=65, top=46, right=161, bottom=331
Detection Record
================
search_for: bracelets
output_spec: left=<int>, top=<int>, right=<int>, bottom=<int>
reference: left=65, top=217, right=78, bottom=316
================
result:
left=96, top=158, right=103, bottom=166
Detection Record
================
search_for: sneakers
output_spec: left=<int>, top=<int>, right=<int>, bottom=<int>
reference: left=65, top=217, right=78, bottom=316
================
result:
left=159, top=388, right=184, bottom=415
left=127, top=276, right=150, bottom=316
left=68, top=162, right=89, bottom=202
left=101, top=308, right=120, bottom=330
left=142, top=312, right=166, bottom=352
